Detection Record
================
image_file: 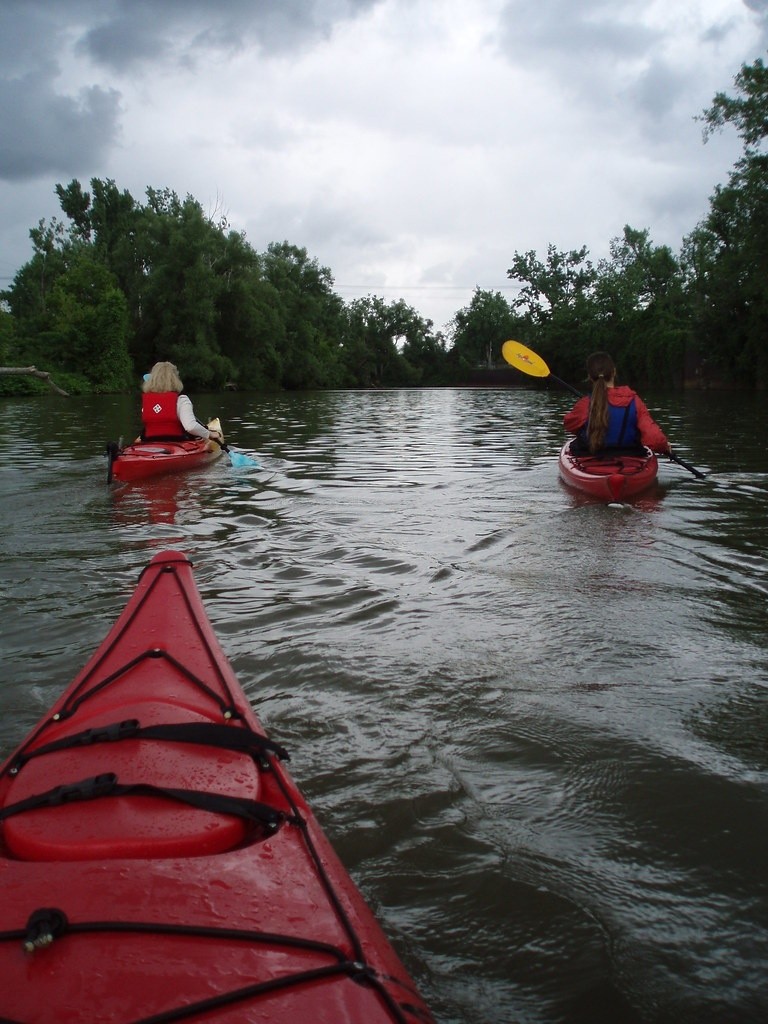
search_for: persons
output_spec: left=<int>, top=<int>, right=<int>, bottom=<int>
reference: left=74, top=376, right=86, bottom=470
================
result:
left=563, top=351, right=673, bottom=457
left=136, top=362, right=221, bottom=443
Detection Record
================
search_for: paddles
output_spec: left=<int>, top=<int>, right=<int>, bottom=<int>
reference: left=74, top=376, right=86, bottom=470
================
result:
left=503, top=337, right=707, bottom=481
left=142, top=375, right=255, bottom=472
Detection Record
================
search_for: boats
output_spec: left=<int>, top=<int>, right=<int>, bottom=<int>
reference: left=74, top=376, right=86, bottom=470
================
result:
left=558, top=435, right=660, bottom=505
left=111, top=416, right=226, bottom=484
